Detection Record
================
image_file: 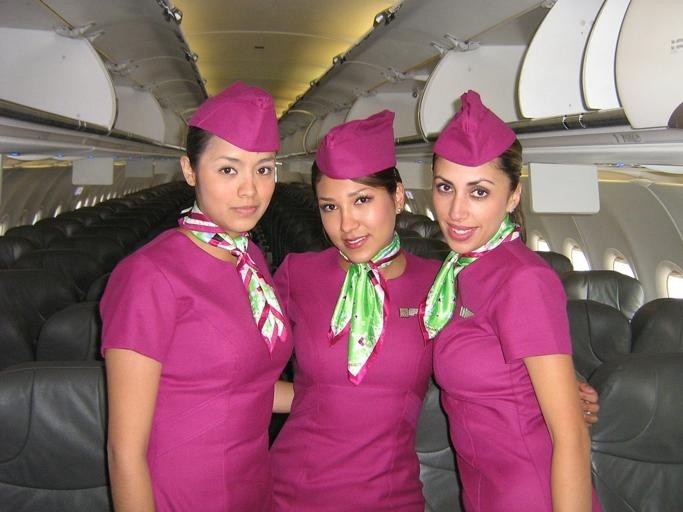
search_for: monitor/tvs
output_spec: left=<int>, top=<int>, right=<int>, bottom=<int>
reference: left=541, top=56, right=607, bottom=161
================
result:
left=288, top=159, right=305, bottom=172
left=395, top=160, right=433, bottom=189
left=155, top=160, right=175, bottom=173
left=124, top=159, right=153, bottom=178
left=528, top=163, right=600, bottom=215
left=71, top=156, right=113, bottom=185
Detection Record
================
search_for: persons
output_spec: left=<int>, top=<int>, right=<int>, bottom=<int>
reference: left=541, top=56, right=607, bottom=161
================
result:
left=420, top=92, right=594, bottom=512
left=271, top=109, right=597, bottom=511
left=99, top=81, right=295, bottom=511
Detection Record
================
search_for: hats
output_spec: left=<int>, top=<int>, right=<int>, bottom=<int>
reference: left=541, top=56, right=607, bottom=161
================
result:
left=186, top=79, right=280, bottom=153
left=430, top=89, right=516, bottom=167
left=315, top=109, right=398, bottom=180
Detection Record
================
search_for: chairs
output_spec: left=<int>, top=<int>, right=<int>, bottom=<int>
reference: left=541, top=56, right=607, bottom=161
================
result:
left=0, top=182, right=683, bottom=510
